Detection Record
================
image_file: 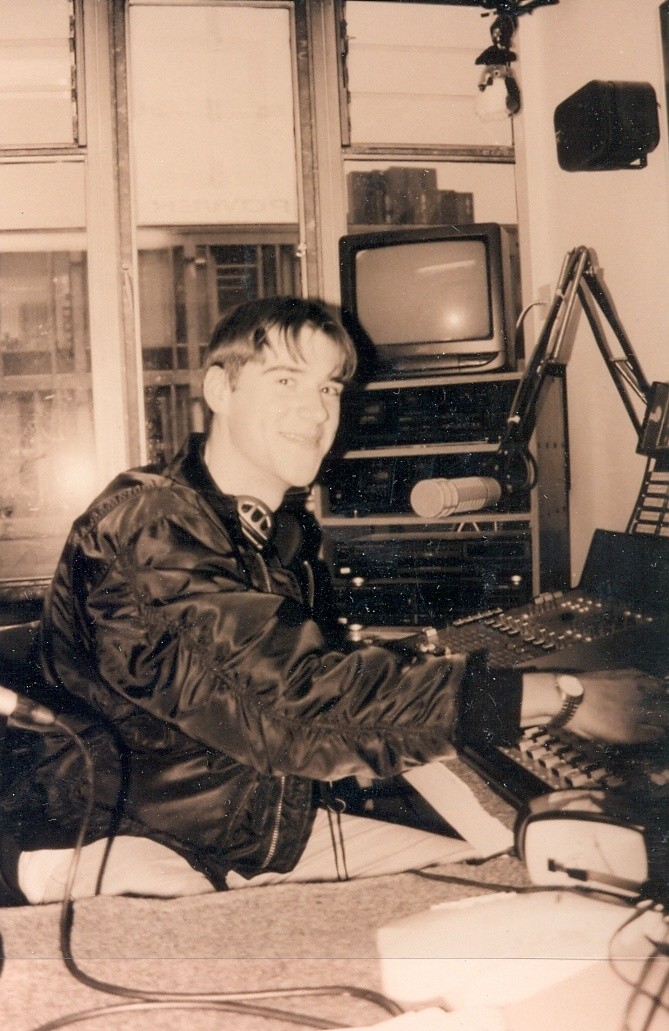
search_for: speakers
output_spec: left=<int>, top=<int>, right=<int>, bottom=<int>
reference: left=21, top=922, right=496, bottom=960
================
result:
left=553, top=81, right=658, bottom=172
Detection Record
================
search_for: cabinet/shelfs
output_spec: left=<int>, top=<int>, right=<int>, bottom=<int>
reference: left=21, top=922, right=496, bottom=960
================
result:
left=313, top=371, right=539, bottom=640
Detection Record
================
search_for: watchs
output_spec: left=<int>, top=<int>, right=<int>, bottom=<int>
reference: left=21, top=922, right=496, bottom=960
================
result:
left=546, top=668, right=585, bottom=729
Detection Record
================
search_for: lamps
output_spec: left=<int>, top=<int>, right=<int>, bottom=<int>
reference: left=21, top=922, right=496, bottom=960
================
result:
left=410, top=244, right=669, bottom=535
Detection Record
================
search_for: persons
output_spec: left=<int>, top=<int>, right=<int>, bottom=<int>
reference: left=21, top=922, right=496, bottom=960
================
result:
left=0, top=294, right=669, bottom=907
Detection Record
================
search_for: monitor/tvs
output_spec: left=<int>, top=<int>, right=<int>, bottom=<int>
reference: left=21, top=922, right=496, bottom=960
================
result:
left=334, top=223, right=518, bottom=381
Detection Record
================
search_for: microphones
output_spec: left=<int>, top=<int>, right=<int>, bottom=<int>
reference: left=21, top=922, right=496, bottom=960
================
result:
left=0, top=687, right=56, bottom=726
left=410, top=476, right=501, bottom=519
